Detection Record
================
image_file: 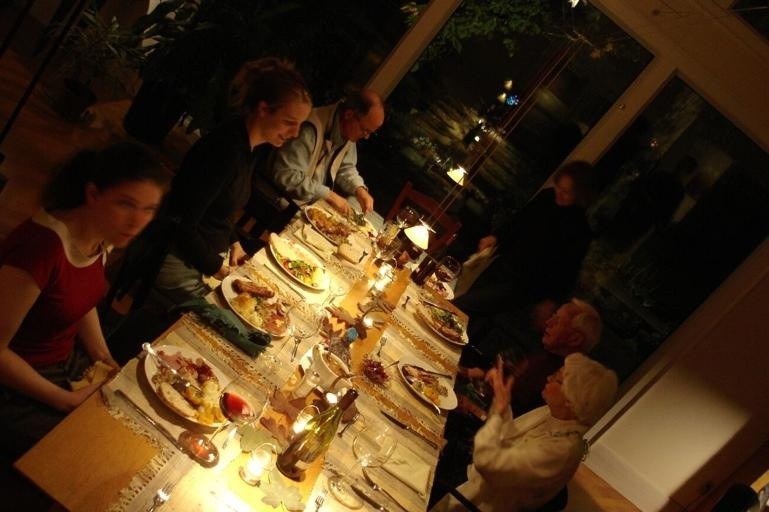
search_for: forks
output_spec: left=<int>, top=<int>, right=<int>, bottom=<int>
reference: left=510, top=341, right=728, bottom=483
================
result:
left=313, top=488, right=329, bottom=512
left=373, top=335, right=388, bottom=358
left=145, top=480, right=176, bottom=511
left=362, top=468, right=409, bottom=512
left=142, top=340, right=203, bottom=397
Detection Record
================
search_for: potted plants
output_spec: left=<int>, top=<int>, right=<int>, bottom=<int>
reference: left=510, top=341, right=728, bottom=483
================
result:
left=41, top=0, right=215, bottom=120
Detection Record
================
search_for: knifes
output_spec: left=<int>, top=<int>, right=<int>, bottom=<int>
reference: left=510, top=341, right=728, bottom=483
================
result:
left=114, top=386, right=184, bottom=453
left=346, top=481, right=389, bottom=512
left=411, top=365, right=452, bottom=379
left=380, top=408, right=438, bottom=449
left=422, top=300, right=458, bottom=316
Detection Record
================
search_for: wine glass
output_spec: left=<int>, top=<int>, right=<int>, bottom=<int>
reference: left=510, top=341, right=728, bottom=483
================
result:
left=327, top=422, right=401, bottom=510
left=322, top=270, right=355, bottom=305
left=358, top=231, right=403, bottom=279
left=181, top=375, right=269, bottom=467
left=423, top=256, right=462, bottom=289
left=253, top=297, right=326, bottom=370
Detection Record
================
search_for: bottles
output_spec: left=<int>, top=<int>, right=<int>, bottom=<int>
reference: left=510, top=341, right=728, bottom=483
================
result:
left=293, top=370, right=320, bottom=401
left=409, top=233, right=460, bottom=287
left=274, top=387, right=357, bottom=479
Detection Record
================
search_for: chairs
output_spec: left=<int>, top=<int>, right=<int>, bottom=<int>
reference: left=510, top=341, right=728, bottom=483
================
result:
left=96, top=224, right=181, bottom=332
left=387, top=181, right=462, bottom=257
left=425, top=478, right=571, bottom=511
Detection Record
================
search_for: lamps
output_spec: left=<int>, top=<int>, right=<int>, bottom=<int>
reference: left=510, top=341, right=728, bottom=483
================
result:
left=358, top=261, right=394, bottom=314
left=403, top=27, right=596, bottom=251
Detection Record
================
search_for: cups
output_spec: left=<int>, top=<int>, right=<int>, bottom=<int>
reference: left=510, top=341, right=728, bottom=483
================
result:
left=386, top=227, right=423, bottom=272
left=311, top=341, right=353, bottom=397
left=393, top=205, right=421, bottom=225
left=239, top=450, right=268, bottom=485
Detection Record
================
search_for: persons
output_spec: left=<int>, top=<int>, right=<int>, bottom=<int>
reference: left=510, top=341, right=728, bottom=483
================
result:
left=427, top=351, right=619, bottom=511
left=121, top=0, right=603, bottom=447
left=2, top=140, right=172, bottom=460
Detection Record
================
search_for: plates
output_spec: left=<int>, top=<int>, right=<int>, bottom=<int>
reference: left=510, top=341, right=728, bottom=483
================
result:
left=411, top=263, right=455, bottom=301
left=218, top=271, right=295, bottom=340
left=305, top=204, right=345, bottom=245
left=144, top=344, right=230, bottom=430
left=396, top=355, right=459, bottom=411
left=263, top=235, right=326, bottom=290
left=331, top=204, right=377, bottom=237
left=412, top=302, right=468, bottom=347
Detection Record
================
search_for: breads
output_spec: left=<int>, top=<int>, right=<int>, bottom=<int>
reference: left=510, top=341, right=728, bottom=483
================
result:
left=159, top=381, right=197, bottom=417
